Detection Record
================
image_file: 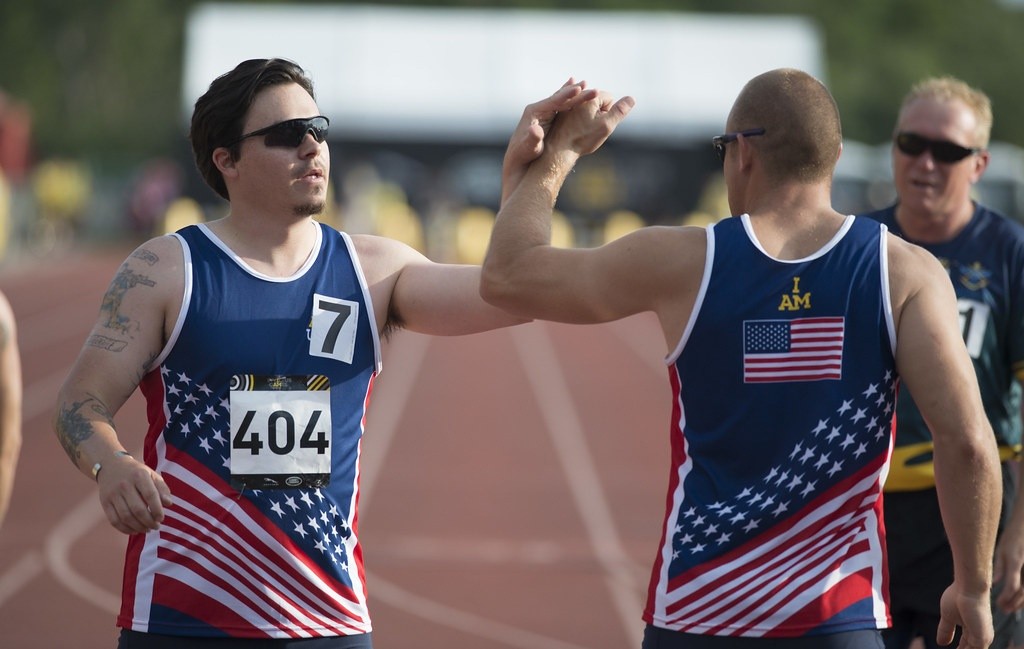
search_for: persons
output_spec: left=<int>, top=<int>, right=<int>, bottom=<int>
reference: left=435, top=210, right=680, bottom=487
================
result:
left=52, top=57, right=601, bottom=649
left=0, top=290, right=25, bottom=527
left=480, top=68, right=1003, bottom=649
left=849, top=76, right=1024, bottom=649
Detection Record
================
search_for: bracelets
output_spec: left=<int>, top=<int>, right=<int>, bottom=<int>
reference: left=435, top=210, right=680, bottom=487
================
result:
left=91, top=451, right=134, bottom=484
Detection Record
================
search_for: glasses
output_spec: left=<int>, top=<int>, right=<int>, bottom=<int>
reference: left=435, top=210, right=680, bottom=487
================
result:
left=896, top=133, right=979, bottom=163
left=712, top=128, right=764, bottom=161
left=224, top=116, right=329, bottom=148
